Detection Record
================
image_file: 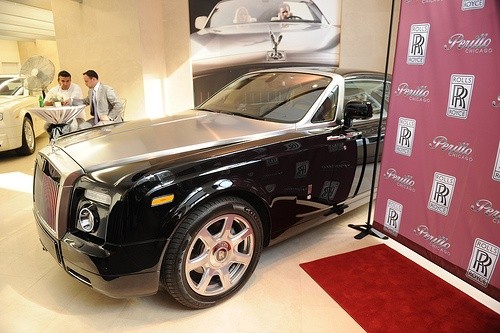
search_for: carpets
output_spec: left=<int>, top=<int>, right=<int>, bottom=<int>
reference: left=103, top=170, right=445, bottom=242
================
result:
left=299, top=243, right=500, bottom=333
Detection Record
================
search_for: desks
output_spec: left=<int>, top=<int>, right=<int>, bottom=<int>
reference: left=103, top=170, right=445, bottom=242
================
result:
left=27, top=105, right=86, bottom=139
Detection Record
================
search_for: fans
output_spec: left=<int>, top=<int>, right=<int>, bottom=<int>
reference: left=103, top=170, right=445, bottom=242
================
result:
left=20, top=56, right=55, bottom=98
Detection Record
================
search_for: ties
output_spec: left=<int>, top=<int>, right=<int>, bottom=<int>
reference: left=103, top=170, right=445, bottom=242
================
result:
left=93, top=91, right=98, bottom=124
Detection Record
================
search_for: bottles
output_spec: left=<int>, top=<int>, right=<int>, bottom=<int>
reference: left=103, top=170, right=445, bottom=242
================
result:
left=39, top=96, right=44, bottom=107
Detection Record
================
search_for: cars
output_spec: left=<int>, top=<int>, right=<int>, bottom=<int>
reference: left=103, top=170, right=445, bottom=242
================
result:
left=189, top=0, right=341, bottom=107
left=31, top=68, right=393, bottom=310
left=0, top=74, right=53, bottom=156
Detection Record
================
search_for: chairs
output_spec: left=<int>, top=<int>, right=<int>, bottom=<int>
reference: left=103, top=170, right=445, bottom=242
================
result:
left=118, top=98, right=127, bottom=120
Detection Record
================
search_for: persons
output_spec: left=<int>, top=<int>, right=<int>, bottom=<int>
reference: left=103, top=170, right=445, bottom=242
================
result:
left=65, top=70, right=123, bottom=131
left=280, top=4, right=291, bottom=19
left=233, top=7, right=251, bottom=24
left=43, top=71, right=83, bottom=140
